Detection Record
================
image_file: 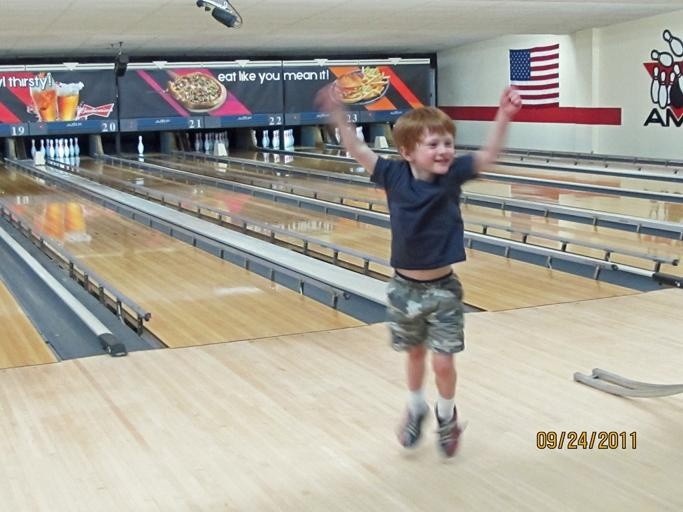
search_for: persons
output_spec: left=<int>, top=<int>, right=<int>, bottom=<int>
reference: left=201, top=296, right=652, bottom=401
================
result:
left=310, top=77, right=523, bottom=459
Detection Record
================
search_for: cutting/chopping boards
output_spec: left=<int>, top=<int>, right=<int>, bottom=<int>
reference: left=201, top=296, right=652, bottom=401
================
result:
left=182, top=71, right=227, bottom=113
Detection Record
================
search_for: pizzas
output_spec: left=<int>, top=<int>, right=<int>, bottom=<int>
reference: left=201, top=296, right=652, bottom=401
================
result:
left=169, top=72, right=223, bottom=109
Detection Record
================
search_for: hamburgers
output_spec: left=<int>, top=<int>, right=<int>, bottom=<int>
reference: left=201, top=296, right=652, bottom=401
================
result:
left=335, top=73, right=364, bottom=102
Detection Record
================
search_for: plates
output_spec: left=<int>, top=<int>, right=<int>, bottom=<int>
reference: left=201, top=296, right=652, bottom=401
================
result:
left=328, top=69, right=391, bottom=106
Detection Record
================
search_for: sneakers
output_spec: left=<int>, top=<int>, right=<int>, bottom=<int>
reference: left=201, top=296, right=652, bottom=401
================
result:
left=434, top=401, right=459, bottom=457
left=398, top=402, right=429, bottom=445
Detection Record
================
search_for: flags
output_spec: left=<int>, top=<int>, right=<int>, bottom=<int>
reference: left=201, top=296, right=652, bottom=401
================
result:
left=507, top=43, right=559, bottom=109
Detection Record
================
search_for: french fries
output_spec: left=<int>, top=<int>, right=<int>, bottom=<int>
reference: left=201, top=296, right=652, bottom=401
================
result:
left=361, top=66, right=389, bottom=98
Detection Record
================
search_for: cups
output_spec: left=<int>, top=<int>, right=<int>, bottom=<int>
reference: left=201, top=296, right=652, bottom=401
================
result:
left=30, top=87, right=57, bottom=123
left=57, top=87, right=79, bottom=122
